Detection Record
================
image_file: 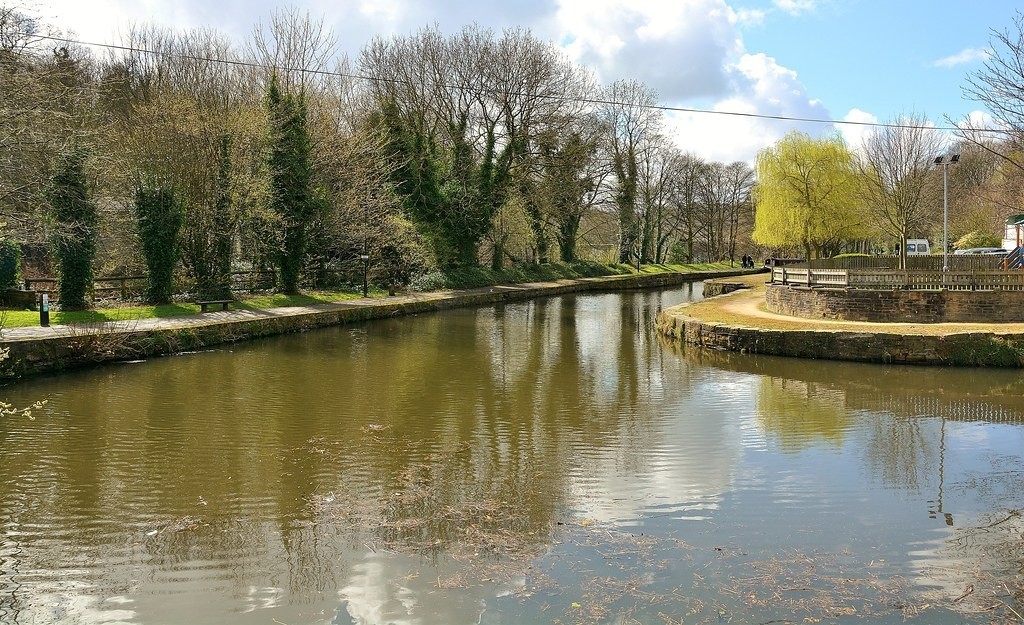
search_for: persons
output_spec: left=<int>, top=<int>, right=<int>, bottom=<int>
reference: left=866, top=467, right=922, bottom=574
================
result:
left=741, top=254, right=755, bottom=269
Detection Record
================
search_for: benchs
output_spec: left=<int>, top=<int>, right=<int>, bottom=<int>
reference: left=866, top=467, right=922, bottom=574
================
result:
left=195, top=299, right=234, bottom=312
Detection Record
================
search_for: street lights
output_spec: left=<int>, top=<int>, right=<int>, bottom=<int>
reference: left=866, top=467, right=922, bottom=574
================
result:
left=933, top=154, right=961, bottom=272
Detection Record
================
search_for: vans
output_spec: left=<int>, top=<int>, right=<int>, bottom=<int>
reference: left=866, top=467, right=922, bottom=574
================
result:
left=905, top=239, right=930, bottom=256
left=963, top=248, right=1005, bottom=254
